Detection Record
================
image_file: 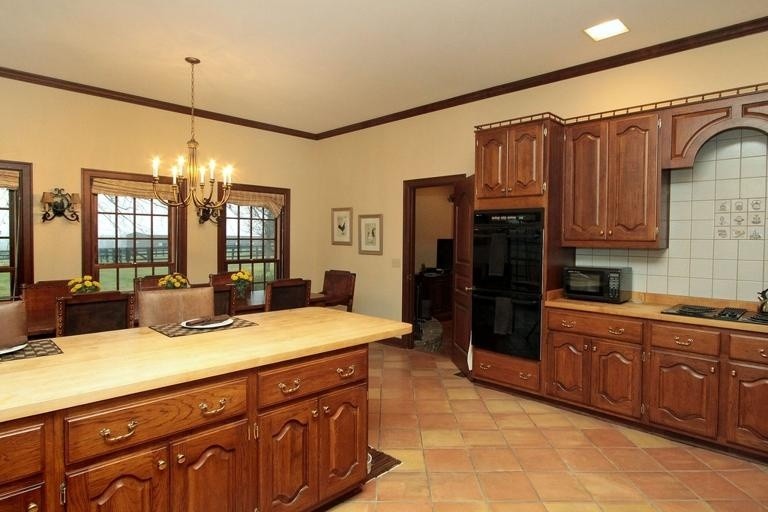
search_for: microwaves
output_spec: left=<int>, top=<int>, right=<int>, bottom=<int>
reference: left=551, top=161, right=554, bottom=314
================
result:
left=560, top=267, right=635, bottom=303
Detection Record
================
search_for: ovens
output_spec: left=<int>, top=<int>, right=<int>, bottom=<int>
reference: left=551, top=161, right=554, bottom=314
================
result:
left=465, top=207, right=542, bottom=361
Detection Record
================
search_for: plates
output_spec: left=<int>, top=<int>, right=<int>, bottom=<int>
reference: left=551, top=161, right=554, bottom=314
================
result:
left=180, top=315, right=234, bottom=330
left=0, top=342, right=29, bottom=355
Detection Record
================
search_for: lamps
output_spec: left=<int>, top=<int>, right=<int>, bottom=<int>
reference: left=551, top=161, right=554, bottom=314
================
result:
left=151, top=56, right=235, bottom=225
left=39, top=186, right=81, bottom=224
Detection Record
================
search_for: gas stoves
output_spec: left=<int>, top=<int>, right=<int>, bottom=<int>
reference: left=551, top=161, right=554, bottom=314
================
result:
left=660, top=303, right=768, bottom=326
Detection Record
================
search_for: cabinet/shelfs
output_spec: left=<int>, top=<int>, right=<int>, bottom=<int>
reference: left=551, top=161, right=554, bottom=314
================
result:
left=473, top=121, right=546, bottom=208
left=258, top=345, right=367, bottom=510
left=647, top=323, right=722, bottom=439
left=470, top=349, right=541, bottom=392
left=562, top=115, right=669, bottom=251
left=0, top=376, right=252, bottom=512
left=545, top=309, right=643, bottom=420
left=726, top=331, right=768, bottom=453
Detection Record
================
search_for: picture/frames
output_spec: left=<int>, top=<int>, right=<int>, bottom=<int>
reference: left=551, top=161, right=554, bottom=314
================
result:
left=330, top=207, right=384, bottom=256
